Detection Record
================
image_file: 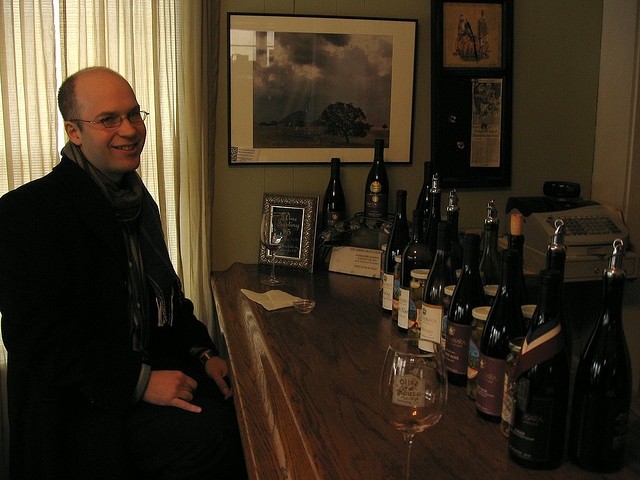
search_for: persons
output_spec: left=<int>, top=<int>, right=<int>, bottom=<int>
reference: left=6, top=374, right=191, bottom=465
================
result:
left=0, top=67, right=247, bottom=480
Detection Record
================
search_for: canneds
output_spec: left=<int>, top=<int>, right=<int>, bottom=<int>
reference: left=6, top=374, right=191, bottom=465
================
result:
left=499, top=335, right=523, bottom=439
left=440, top=284, right=457, bottom=352
left=464, top=306, right=492, bottom=401
left=481, top=283, right=500, bottom=308
left=453, top=267, right=486, bottom=280
left=519, top=302, right=538, bottom=336
left=377, top=244, right=388, bottom=311
left=405, top=267, right=429, bottom=348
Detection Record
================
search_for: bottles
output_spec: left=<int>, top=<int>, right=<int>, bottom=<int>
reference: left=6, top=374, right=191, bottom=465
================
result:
left=479, top=217, right=502, bottom=285
left=417, top=162, right=436, bottom=223
left=425, top=189, right=441, bottom=260
left=508, top=269, right=573, bottom=470
left=323, top=158, right=346, bottom=229
left=529, top=245, right=572, bottom=375
left=446, top=207, right=464, bottom=260
left=381, top=190, right=410, bottom=308
left=568, top=268, right=632, bottom=473
left=364, top=139, right=389, bottom=220
left=476, top=249, right=525, bottom=422
left=507, top=235, right=529, bottom=304
left=444, top=226, right=486, bottom=386
left=398, top=209, right=431, bottom=334
left=416, top=221, right=457, bottom=353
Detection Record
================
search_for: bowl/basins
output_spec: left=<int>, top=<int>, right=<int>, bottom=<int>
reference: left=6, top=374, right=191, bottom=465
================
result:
left=539, top=178, right=580, bottom=201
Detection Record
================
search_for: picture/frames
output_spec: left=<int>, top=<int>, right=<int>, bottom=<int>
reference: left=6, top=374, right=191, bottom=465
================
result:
left=431, top=0, right=514, bottom=191
left=259, top=195, right=319, bottom=268
left=228, top=13, right=419, bottom=167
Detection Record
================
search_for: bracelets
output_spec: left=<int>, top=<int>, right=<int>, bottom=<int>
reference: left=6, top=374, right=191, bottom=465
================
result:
left=198, top=351, right=219, bottom=370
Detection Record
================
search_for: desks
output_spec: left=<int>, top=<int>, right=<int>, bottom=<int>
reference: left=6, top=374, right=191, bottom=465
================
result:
left=208, top=262, right=535, bottom=478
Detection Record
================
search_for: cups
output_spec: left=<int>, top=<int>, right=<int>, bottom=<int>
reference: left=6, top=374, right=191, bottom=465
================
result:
left=294, top=300, right=316, bottom=314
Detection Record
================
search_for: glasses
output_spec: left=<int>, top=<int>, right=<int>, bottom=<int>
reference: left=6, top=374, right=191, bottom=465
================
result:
left=65, top=110, right=150, bottom=129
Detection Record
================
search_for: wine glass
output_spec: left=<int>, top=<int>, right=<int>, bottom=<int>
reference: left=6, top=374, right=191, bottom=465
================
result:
left=259, top=212, right=288, bottom=287
left=378, top=339, right=448, bottom=480
